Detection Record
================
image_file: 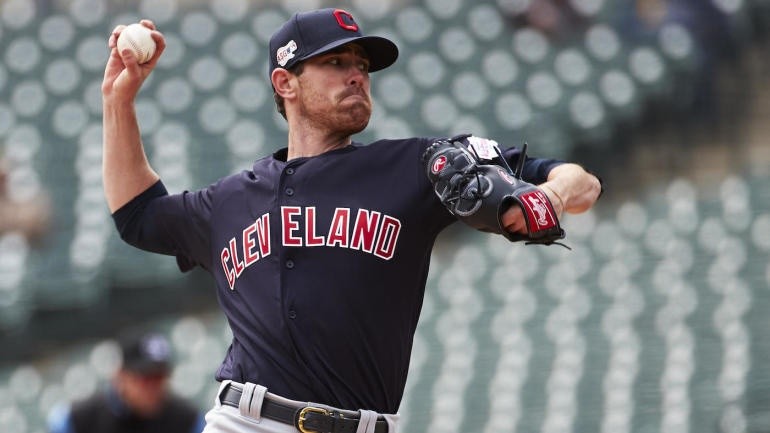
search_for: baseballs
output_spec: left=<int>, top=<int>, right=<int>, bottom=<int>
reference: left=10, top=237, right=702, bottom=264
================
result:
left=117, top=23, right=157, bottom=65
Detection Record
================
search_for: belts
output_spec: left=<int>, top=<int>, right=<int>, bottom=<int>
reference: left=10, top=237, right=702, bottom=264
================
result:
left=219, top=383, right=389, bottom=433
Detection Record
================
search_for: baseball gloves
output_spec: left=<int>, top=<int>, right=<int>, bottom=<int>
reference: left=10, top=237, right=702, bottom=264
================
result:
left=419, top=140, right=565, bottom=244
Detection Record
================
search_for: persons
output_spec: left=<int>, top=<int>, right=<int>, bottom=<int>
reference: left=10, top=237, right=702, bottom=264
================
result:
left=100, top=8, right=604, bottom=433
left=57, top=326, right=207, bottom=433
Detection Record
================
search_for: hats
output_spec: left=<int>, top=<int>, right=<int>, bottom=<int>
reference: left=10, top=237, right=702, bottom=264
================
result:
left=120, top=332, right=179, bottom=378
left=268, top=8, right=399, bottom=85
